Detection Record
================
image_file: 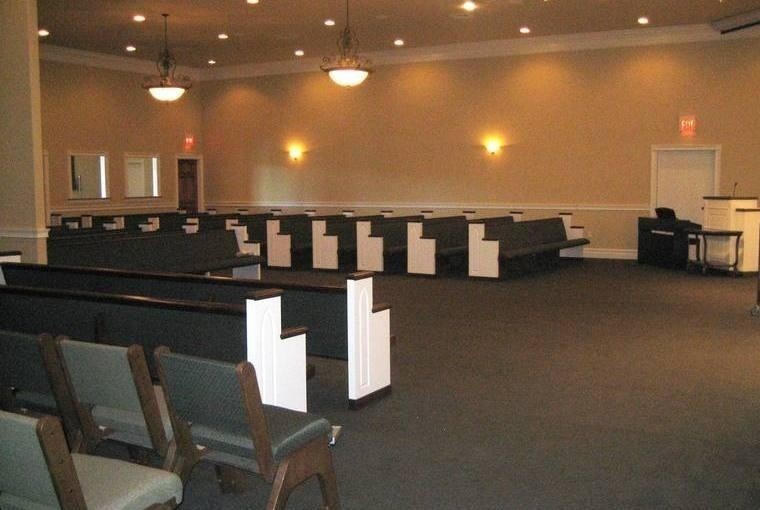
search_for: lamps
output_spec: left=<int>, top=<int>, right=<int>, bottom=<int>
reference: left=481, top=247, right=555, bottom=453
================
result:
left=142, top=13, right=192, bottom=101
left=321, top=0, right=375, bottom=87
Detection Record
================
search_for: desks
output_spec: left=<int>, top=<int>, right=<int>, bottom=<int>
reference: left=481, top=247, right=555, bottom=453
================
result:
left=689, top=228, right=742, bottom=276
